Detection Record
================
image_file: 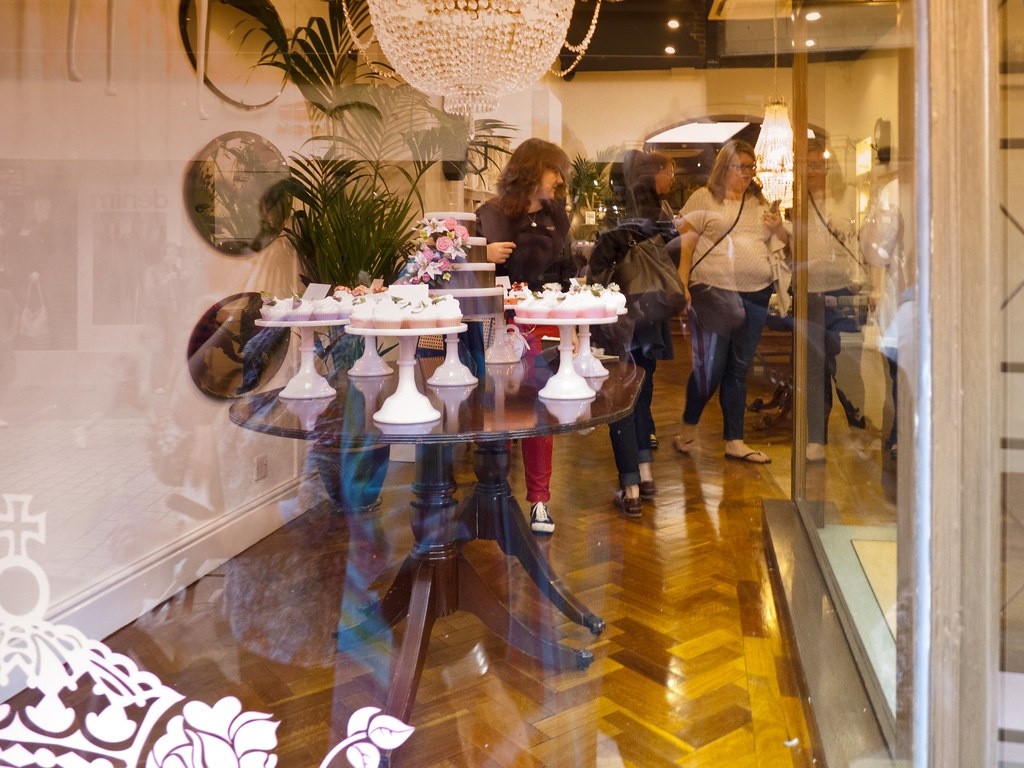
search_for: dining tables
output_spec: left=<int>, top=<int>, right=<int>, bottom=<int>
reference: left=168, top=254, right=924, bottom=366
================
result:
left=229, top=355, right=646, bottom=768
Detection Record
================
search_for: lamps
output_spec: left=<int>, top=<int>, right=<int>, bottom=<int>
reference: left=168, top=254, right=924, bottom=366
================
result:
left=341, top=0, right=602, bottom=116
left=752, top=0, right=793, bottom=209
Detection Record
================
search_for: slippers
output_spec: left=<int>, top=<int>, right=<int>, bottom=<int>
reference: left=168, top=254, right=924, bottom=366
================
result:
left=724, top=450, right=772, bottom=464
left=674, top=432, right=693, bottom=455
left=806, top=457, right=828, bottom=466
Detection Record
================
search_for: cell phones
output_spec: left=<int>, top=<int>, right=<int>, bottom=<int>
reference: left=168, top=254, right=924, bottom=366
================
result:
left=771, top=201, right=782, bottom=215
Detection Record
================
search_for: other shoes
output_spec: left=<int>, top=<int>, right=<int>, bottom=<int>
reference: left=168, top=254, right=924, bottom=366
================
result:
left=651, top=439, right=659, bottom=447
left=614, top=489, right=643, bottom=517
left=639, top=480, right=655, bottom=499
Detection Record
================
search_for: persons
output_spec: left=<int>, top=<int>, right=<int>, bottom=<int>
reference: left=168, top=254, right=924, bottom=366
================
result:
left=804, top=138, right=875, bottom=467
left=862, top=184, right=910, bottom=321
left=588, top=146, right=679, bottom=523
left=468, top=137, right=575, bottom=537
left=169, top=133, right=788, bottom=768
left=674, top=138, right=790, bottom=464
left=633, top=152, right=680, bottom=450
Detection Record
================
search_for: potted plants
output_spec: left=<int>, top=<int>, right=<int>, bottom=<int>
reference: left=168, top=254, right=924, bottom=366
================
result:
left=215, top=1, right=519, bottom=514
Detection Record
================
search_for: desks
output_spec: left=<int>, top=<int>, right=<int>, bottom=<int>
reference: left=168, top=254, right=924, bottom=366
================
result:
left=747, top=305, right=867, bottom=445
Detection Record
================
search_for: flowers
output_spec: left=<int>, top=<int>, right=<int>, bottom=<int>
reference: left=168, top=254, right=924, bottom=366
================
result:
left=404, top=215, right=472, bottom=287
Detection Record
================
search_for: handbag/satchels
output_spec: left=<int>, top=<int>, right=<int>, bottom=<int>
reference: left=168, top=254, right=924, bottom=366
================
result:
left=592, top=227, right=686, bottom=338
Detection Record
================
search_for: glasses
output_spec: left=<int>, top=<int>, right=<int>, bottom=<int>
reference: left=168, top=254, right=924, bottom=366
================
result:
left=806, top=162, right=822, bottom=170
left=662, top=170, right=674, bottom=177
left=729, top=163, right=757, bottom=171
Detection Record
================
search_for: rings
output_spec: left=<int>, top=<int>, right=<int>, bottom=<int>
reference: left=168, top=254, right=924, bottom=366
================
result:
left=771, top=214, right=777, bottom=221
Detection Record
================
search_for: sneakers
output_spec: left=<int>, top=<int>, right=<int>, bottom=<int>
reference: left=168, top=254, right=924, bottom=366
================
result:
left=529, top=502, right=555, bottom=534
